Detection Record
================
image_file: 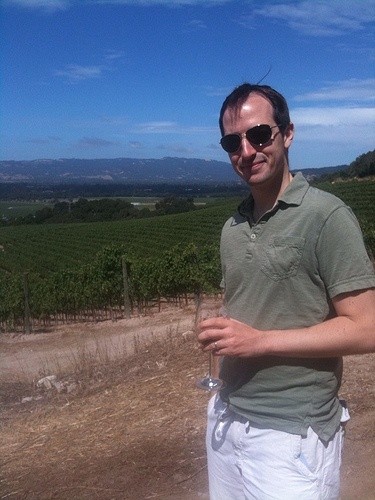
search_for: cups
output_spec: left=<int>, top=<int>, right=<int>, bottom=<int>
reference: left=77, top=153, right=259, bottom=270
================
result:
left=194, top=296, right=228, bottom=389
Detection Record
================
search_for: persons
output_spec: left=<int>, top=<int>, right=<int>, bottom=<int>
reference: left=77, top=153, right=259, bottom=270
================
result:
left=192, top=82, right=375, bottom=500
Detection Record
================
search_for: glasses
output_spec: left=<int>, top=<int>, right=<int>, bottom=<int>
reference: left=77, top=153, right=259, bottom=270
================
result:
left=220, top=124, right=282, bottom=154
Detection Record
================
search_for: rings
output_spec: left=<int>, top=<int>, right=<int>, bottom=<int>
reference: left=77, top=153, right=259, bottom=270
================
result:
left=214, top=342, right=219, bottom=349
